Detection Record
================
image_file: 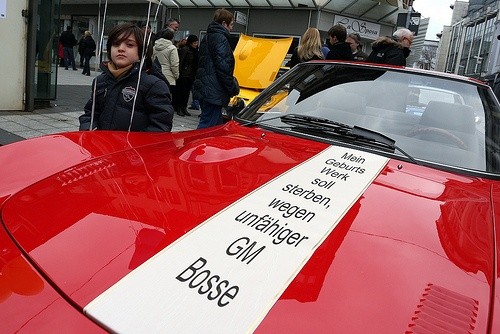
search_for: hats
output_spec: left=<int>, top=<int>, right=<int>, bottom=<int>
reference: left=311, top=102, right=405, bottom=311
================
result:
left=187, top=34, right=199, bottom=43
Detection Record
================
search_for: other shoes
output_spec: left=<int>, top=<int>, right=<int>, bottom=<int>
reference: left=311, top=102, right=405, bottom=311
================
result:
left=177, top=110, right=185, bottom=117
left=188, top=106, right=199, bottom=110
left=73, top=68, right=77, bottom=70
left=87, top=74, right=90, bottom=76
left=65, top=68, right=68, bottom=70
left=183, top=109, right=191, bottom=116
left=82, top=72, right=85, bottom=75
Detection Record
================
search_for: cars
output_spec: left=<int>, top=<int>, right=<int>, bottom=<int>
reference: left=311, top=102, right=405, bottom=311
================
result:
left=0, top=60, right=500, bottom=334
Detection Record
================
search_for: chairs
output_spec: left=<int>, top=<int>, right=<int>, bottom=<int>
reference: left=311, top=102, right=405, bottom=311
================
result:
left=368, top=74, right=408, bottom=112
left=417, top=100, right=479, bottom=153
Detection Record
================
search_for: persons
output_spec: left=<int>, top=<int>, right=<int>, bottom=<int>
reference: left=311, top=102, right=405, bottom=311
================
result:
left=77, top=22, right=173, bottom=134
left=191, top=8, right=241, bottom=130
left=53, top=19, right=414, bottom=127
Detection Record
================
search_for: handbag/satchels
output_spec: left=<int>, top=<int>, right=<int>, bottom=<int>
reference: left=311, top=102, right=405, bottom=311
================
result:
left=92, top=51, right=96, bottom=56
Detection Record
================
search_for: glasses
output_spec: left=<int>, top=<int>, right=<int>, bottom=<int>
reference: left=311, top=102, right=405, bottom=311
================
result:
left=402, top=36, right=413, bottom=45
